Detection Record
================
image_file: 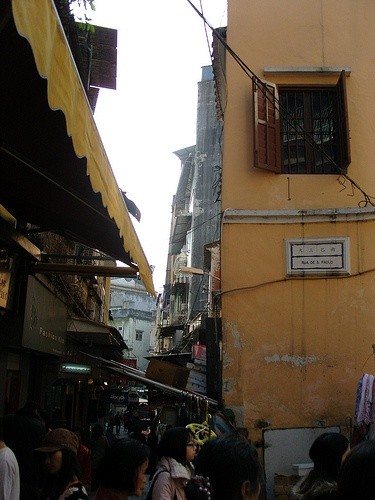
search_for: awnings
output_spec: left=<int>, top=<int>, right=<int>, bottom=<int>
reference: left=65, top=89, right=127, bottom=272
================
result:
left=0, top=0, right=154, bottom=297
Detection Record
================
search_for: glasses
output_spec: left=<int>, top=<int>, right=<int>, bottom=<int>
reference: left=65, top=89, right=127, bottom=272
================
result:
left=187, top=441, right=197, bottom=448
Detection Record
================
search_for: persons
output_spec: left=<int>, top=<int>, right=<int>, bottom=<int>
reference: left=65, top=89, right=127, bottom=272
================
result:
left=0, top=411, right=264, bottom=500
left=291, top=432, right=375, bottom=500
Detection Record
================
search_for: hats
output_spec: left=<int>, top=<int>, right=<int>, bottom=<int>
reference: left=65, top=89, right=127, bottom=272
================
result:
left=33, top=428, right=79, bottom=456
